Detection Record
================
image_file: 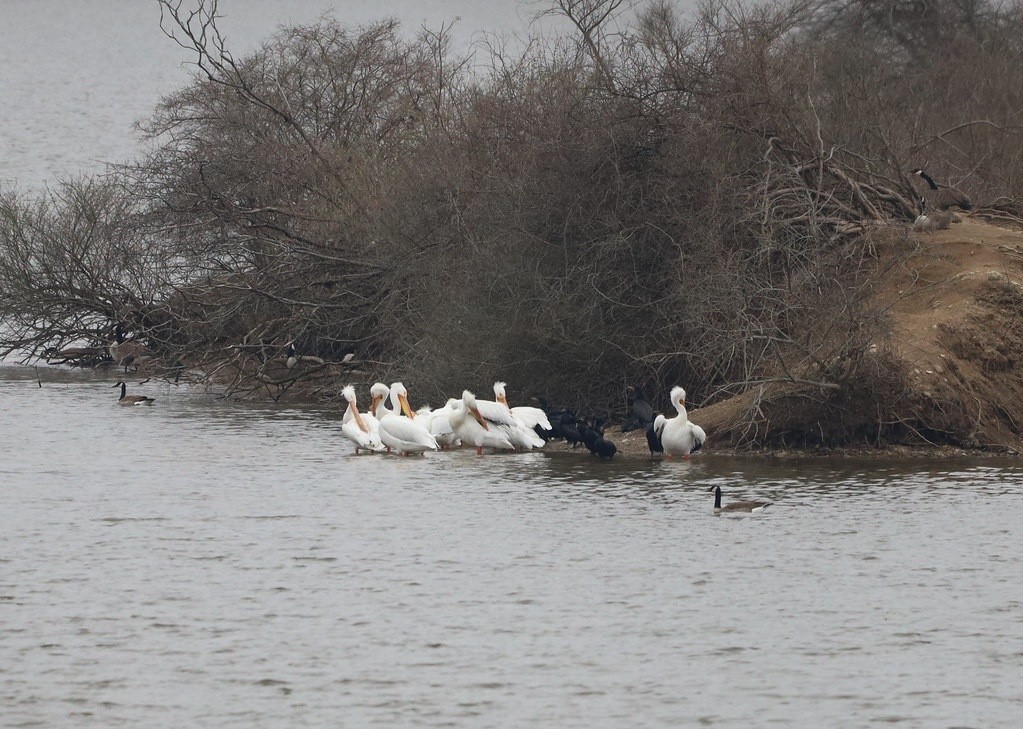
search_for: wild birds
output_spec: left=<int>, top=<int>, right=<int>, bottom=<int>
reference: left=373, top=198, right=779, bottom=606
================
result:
left=83, top=319, right=301, bottom=374
left=111, top=381, right=157, bottom=407
left=340, top=380, right=707, bottom=460
left=703, top=485, right=775, bottom=516
